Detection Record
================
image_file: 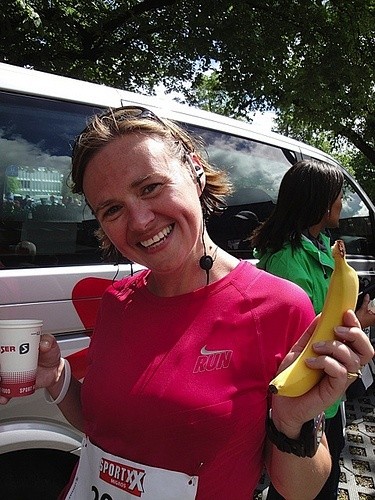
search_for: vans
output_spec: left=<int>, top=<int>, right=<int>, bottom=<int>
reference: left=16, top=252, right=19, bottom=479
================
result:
left=0, top=62, right=375, bottom=500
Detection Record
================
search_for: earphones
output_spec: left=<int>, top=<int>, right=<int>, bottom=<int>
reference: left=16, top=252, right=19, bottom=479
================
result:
left=194, top=165, right=203, bottom=181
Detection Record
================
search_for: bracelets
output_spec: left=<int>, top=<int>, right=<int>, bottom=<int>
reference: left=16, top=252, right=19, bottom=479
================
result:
left=43, top=357, right=71, bottom=405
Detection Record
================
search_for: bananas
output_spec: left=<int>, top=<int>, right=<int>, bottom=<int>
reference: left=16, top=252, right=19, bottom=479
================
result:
left=268, top=240, right=359, bottom=398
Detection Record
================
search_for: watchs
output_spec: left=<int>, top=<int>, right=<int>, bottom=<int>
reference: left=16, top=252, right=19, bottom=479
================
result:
left=264, top=408, right=326, bottom=458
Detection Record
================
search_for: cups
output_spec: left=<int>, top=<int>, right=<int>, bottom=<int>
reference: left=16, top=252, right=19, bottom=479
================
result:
left=0, top=320, right=44, bottom=398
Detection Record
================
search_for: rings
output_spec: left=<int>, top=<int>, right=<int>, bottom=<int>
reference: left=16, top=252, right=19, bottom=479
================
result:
left=347, top=366, right=365, bottom=381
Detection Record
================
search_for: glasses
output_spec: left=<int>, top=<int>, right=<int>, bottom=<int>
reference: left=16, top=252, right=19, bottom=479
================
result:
left=72, top=106, right=187, bottom=168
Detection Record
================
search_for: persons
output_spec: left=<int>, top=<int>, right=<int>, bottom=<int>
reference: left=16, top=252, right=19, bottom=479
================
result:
left=0, top=105, right=375, bottom=500
left=250, top=158, right=375, bottom=500
